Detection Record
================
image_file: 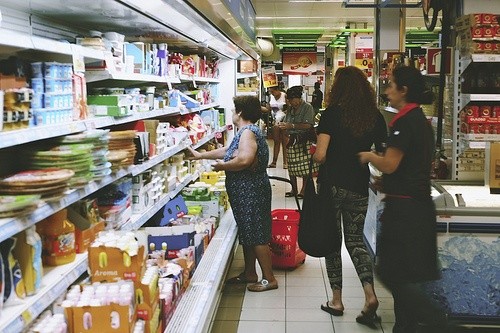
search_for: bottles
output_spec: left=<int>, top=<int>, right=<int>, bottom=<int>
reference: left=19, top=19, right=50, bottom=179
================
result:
left=32, top=231, right=158, bottom=333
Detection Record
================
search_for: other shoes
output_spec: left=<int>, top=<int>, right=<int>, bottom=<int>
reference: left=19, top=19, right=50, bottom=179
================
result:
left=247, top=279, right=278, bottom=291
left=229, top=272, right=259, bottom=284
left=285, top=191, right=298, bottom=197
left=297, top=194, right=304, bottom=199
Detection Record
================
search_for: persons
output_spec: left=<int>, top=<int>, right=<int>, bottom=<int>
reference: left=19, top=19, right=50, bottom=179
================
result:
left=358, top=65, right=438, bottom=317
left=312, top=65, right=387, bottom=326
left=276, top=85, right=319, bottom=198
left=311, top=82, right=324, bottom=115
left=261, top=85, right=289, bottom=168
left=186, top=95, right=278, bottom=292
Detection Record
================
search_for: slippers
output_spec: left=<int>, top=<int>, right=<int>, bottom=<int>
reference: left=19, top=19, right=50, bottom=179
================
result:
left=355, top=310, right=382, bottom=324
left=321, top=301, right=343, bottom=316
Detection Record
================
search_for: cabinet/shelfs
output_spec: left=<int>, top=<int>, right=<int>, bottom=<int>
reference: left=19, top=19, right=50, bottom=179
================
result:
left=325, top=13, right=500, bottom=194
left=0, top=0, right=270, bottom=333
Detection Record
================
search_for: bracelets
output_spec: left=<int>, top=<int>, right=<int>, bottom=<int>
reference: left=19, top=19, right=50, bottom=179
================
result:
left=292, top=122, right=296, bottom=129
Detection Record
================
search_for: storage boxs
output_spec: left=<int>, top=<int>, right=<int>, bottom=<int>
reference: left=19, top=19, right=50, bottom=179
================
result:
left=268, top=175, right=306, bottom=272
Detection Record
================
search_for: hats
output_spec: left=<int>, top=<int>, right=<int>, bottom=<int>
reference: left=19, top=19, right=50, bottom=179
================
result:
left=286, top=85, right=303, bottom=99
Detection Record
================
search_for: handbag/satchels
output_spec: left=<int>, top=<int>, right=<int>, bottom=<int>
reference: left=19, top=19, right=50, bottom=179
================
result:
left=298, top=153, right=338, bottom=257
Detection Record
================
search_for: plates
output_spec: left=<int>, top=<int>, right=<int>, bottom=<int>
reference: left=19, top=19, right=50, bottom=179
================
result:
left=0, top=131, right=137, bottom=218
